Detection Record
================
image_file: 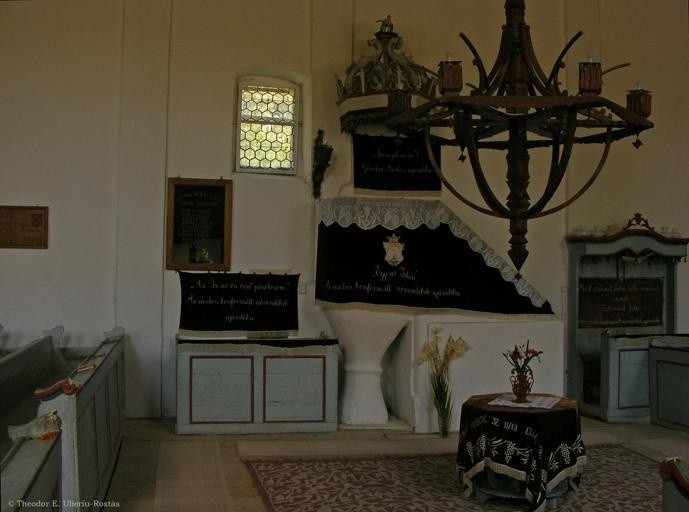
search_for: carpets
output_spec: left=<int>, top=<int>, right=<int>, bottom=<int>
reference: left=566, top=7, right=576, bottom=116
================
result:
left=240, top=440, right=688, bottom=512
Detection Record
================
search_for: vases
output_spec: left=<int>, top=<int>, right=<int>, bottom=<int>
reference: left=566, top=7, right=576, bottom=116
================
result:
left=438, top=413, right=454, bottom=436
left=509, top=370, right=534, bottom=403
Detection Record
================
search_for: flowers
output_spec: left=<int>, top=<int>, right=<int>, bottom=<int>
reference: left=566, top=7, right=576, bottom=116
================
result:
left=502, top=337, right=546, bottom=375
left=413, top=325, right=476, bottom=426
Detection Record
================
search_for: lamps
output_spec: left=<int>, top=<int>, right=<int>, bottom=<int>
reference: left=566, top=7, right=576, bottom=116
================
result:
left=408, top=0, right=655, bottom=278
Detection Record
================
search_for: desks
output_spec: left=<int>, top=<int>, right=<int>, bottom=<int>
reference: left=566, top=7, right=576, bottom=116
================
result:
left=458, top=392, right=589, bottom=511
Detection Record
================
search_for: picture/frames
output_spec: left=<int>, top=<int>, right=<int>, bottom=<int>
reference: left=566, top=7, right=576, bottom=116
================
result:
left=164, top=175, right=235, bottom=270
